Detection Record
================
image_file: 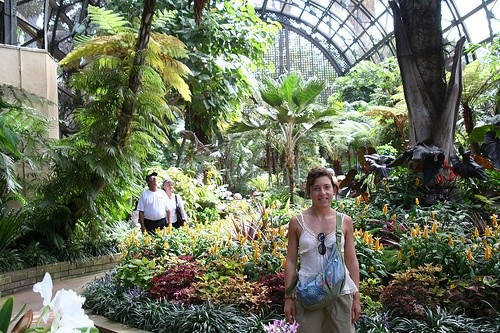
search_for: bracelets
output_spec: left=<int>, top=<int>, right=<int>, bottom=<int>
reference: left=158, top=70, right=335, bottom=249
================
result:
left=284, top=297, right=292, bottom=300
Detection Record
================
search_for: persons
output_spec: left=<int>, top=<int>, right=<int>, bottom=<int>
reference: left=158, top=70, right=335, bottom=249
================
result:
left=138, top=172, right=174, bottom=234
left=161, top=179, right=187, bottom=228
left=284, top=168, right=361, bottom=333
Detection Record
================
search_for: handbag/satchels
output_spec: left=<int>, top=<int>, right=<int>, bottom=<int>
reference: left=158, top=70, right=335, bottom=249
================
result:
left=175, top=195, right=184, bottom=226
left=296, top=242, right=346, bottom=311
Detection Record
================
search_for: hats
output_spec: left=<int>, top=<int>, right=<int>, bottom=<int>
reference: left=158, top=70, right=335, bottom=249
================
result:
left=146, top=172, right=158, bottom=182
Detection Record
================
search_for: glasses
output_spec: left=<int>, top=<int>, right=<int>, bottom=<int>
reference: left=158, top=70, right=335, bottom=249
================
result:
left=317, top=232, right=326, bottom=255
